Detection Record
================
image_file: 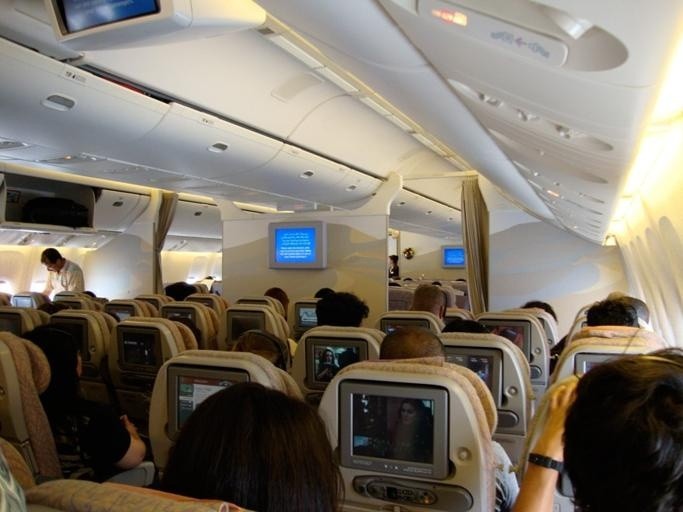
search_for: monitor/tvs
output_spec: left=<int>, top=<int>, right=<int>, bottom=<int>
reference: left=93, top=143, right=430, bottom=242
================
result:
left=268, top=220, right=327, bottom=270
left=442, top=245, right=468, bottom=269
left=48, top=0, right=162, bottom=34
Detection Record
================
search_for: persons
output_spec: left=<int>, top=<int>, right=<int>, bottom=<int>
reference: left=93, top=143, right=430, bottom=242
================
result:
left=0, top=248, right=683, bottom=512
